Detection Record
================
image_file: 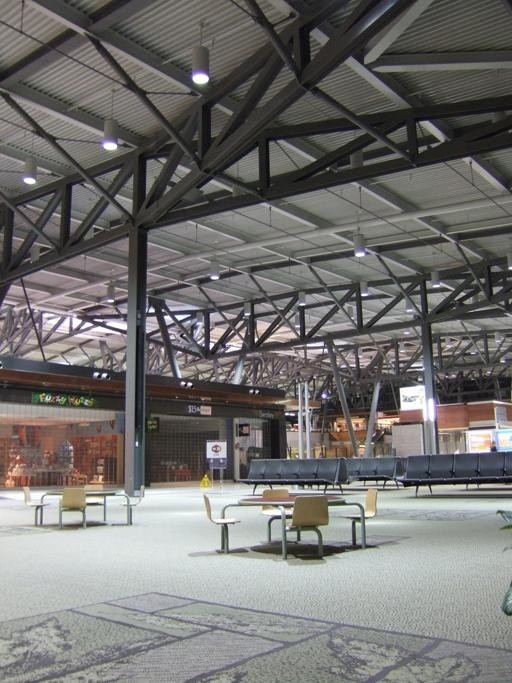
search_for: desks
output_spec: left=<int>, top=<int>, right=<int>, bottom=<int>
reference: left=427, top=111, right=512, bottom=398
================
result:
left=216, top=497, right=366, bottom=553
left=41, top=491, right=129, bottom=526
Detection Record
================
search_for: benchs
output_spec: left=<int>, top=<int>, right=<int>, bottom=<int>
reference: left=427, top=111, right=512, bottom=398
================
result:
left=236, top=452, right=512, bottom=497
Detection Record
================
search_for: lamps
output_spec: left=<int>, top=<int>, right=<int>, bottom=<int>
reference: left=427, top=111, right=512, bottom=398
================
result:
left=353, top=228, right=367, bottom=259
left=103, top=90, right=119, bottom=152
left=192, top=24, right=211, bottom=85
left=210, top=253, right=221, bottom=282
left=107, top=286, right=114, bottom=303
left=195, top=256, right=510, bottom=404
left=22, top=134, right=38, bottom=186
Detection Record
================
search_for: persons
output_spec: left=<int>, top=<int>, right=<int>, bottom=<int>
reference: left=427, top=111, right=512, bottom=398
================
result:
left=490, top=441, right=497, bottom=452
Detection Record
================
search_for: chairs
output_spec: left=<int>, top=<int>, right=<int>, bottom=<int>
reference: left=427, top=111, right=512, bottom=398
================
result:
left=61, top=488, right=86, bottom=527
left=87, top=485, right=105, bottom=505
left=204, top=495, right=240, bottom=554
left=122, top=485, right=144, bottom=525
left=340, top=489, right=378, bottom=549
left=287, top=495, right=329, bottom=559
left=23, top=487, right=46, bottom=526
left=262, top=489, right=300, bottom=543
